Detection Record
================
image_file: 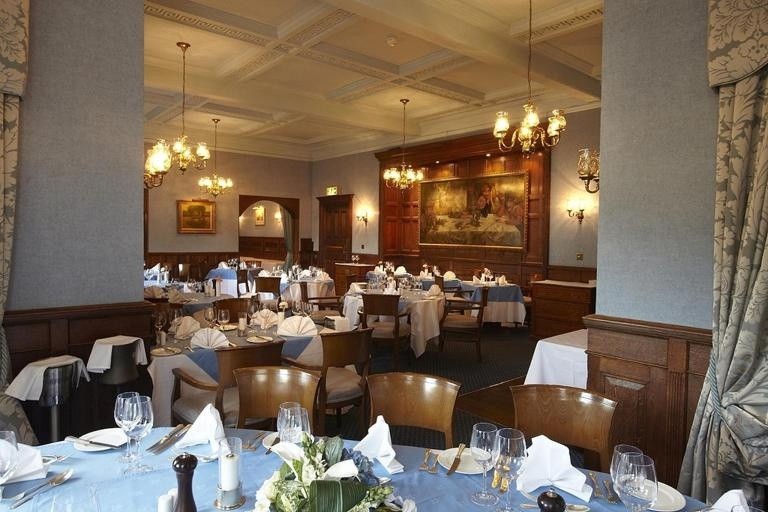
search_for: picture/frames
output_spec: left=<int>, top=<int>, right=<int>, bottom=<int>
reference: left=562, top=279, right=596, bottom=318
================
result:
left=177, top=200, right=217, bottom=234
left=255, top=208, right=265, bottom=225
left=418, top=171, right=528, bottom=252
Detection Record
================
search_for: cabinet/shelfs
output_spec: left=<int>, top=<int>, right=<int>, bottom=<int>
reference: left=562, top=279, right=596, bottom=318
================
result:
left=531, top=278, right=595, bottom=338
left=334, top=262, right=375, bottom=296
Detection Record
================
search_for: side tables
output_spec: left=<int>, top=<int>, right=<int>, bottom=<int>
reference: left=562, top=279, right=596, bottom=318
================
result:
left=523, top=329, right=586, bottom=390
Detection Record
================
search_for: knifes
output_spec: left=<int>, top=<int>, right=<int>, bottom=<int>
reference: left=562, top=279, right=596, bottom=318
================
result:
left=254, top=336, right=271, bottom=343
left=152, top=424, right=192, bottom=455
left=446, top=443, right=466, bottom=475
left=64, top=436, right=121, bottom=449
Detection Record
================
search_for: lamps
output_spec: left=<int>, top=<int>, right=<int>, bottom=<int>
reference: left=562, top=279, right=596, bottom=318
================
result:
left=143, top=137, right=173, bottom=190
left=198, top=173, right=234, bottom=198
left=493, top=0, right=567, bottom=159
left=577, top=146, right=600, bottom=194
left=172, top=42, right=210, bottom=175
left=566, top=199, right=590, bottom=224
left=356, top=206, right=368, bottom=222
left=382, top=100, right=423, bottom=193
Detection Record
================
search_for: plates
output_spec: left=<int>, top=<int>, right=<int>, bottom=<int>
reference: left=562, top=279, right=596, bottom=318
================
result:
left=262, top=433, right=314, bottom=450
left=246, top=336, right=273, bottom=343
left=149, top=348, right=181, bottom=356
left=437, top=448, right=493, bottom=475
left=649, top=482, right=686, bottom=512
left=218, top=325, right=236, bottom=330
left=73, top=428, right=126, bottom=451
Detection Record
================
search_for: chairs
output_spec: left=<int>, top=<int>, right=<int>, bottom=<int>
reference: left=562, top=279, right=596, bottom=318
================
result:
left=212, top=298, right=253, bottom=325
left=236, top=269, right=255, bottom=299
left=347, top=273, right=359, bottom=292
left=365, top=372, right=463, bottom=449
left=282, top=328, right=375, bottom=437
left=233, top=365, right=322, bottom=435
left=435, top=275, right=465, bottom=315
left=178, top=264, right=190, bottom=282
left=171, top=340, right=285, bottom=430
left=520, top=295, right=529, bottom=325
left=510, top=385, right=619, bottom=474
left=245, top=260, right=261, bottom=269
left=300, top=281, right=343, bottom=316
left=255, top=277, right=294, bottom=316
left=357, top=294, right=413, bottom=371
left=439, top=286, right=489, bottom=362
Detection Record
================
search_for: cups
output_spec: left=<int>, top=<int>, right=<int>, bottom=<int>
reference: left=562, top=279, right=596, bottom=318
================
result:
left=165, top=270, right=169, bottom=283
left=352, top=255, right=354, bottom=264
left=152, top=309, right=167, bottom=350
left=312, top=272, right=315, bottom=280
left=492, top=428, right=527, bottom=512
left=495, top=274, right=500, bottom=283
left=258, top=302, right=271, bottom=336
left=168, top=308, right=183, bottom=350
left=309, top=266, right=313, bottom=272
left=276, top=402, right=303, bottom=438
left=470, top=423, right=499, bottom=507
left=699, top=508, right=729, bottom=512
left=227, top=258, right=237, bottom=269
left=489, top=274, right=493, bottom=282
left=413, top=290, right=421, bottom=300
left=186, top=278, right=197, bottom=301
left=144, top=269, right=154, bottom=286
left=610, top=444, right=642, bottom=483
left=295, top=274, right=299, bottom=280
left=0, top=431, right=17, bottom=486
left=158, top=273, right=162, bottom=285
left=217, top=436, right=242, bottom=489
left=278, top=266, right=283, bottom=273
left=273, top=267, right=276, bottom=272
left=356, top=255, right=358, bottom=264
left=122, top=396, right=153, bottom=477
left=114, top=392, right=139, bottom=465
left=314, top=270, right=318, bottom=280
left=731, top=505, right=753, bottom=512
left=252, top=260, right=256, bottom=268
left=612, top=452, right=639, bottom=512
left=247, top=302, right=260, bottom=331
left=289, top=271, right=292, bottom=279
left=217, top=310, right=229, bottom=332
left=277, top=408, right=310, bottom=446
left=204, top=306, right=217, bottom=330
left=613, top=453, right=658, bottom=512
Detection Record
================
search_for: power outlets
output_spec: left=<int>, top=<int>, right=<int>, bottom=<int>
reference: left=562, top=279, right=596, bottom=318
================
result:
left=576, top=253, right=583, bottom=260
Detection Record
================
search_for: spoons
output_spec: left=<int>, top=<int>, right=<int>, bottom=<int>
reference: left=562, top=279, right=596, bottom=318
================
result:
left=520, top=504, right=591, bottom=512
left=590, top=472, right=605, bottom=496
left=7, top=470, right=75, bottom=509
left=419, top=449, right=431, bottom=471
left=428, top=454, right=439, bottom=475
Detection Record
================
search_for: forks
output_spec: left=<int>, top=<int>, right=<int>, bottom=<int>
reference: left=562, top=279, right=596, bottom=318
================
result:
left=144, top=424, right=183, bottom=453
left=9, top=481, right=50, bottom=502
left=56, top=452, right=74, bottom=463
left=603, top=480, right=619, bottom=505
